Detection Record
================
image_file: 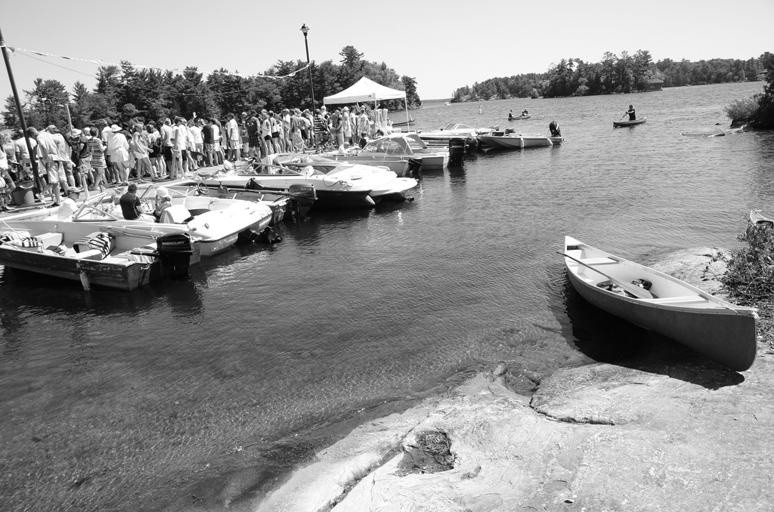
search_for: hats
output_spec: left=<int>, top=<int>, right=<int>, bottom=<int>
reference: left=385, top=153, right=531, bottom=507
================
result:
left=108, top=124, right=122, bottom=133
left=155, top=186, right=169, bottom=198
left=70, top=128, right=82, bottom=137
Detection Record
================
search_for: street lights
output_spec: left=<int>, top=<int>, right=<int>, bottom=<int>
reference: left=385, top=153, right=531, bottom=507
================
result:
left=300, top=23, right=316, bottom=116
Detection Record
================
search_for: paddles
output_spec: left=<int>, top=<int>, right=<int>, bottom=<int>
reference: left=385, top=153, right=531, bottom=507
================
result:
left=556, top=251, right=652, bottom=299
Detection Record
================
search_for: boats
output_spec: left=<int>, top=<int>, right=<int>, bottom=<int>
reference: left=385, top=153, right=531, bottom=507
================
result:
left=612, top=116, right=647, bottom=128
left=513, top=114, right=531, bottom=119
left=562, top=234, right=759, bottom=373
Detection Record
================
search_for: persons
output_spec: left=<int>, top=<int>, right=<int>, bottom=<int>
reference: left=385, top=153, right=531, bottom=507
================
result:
left=522, top=109, right=528, bottom=116
left=509, top=110, right=514, bottom=118
left=626, top=105, right=635, bottom=120
left=550, top=121, right=561, bottom=137
